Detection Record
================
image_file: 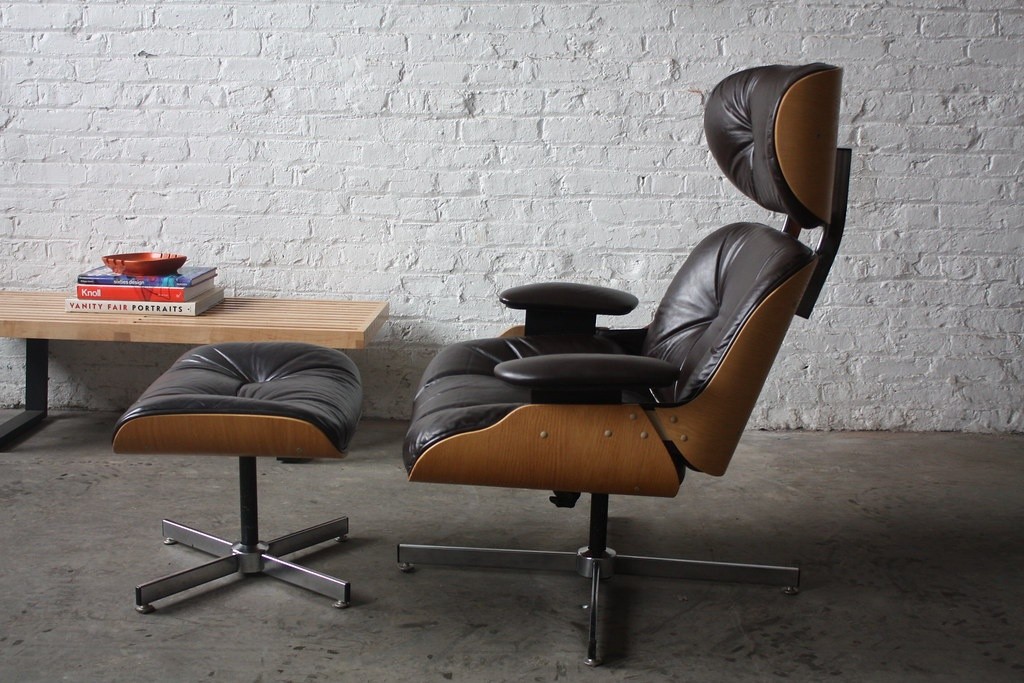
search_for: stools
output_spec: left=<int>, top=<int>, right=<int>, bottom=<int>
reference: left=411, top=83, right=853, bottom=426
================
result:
left=112, top=341, right=364, bottom=614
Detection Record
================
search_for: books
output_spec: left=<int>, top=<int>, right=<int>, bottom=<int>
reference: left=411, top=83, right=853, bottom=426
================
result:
left=64, top=264, right=225, bottom=316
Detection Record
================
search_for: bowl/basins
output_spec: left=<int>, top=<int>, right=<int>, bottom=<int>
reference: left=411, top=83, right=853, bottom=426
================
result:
left=102, top=252, right=187, bottom=276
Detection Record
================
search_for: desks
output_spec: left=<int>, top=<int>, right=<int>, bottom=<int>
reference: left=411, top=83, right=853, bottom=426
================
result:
left=1, top=290, right=390, bottom=463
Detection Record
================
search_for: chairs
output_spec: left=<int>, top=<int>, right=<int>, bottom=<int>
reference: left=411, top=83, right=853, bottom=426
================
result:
left=397, top=63, right=857, bottom=667
